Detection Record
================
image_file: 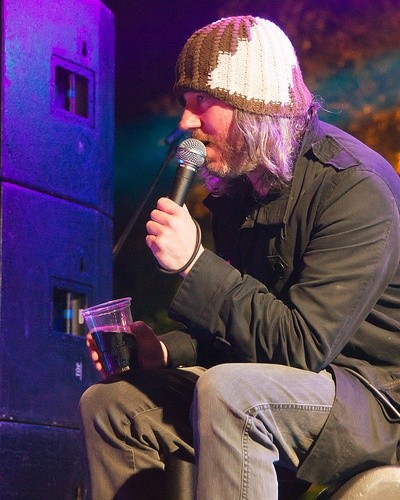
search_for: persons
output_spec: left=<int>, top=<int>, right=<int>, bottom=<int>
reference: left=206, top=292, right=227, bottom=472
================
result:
left=80, top=14, right=400, bottom=500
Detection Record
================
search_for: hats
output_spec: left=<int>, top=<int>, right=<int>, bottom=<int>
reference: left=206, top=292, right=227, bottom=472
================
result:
left=174, top=16, right=312, bottom=117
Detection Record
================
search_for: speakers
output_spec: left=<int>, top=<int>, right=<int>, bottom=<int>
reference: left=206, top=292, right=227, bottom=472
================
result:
left=0, top=182, right=114, bottom=422
left=0, top=422, right=90, bottom=500
left=0, top=0, right=117, bottom=220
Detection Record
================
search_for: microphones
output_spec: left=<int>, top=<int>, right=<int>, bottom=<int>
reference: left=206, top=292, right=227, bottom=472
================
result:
left=169, top=138, right=206, bottom=207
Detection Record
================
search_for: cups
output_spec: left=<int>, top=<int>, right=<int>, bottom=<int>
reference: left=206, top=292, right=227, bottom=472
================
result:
left=81, top=297, right=139, bottom=378
